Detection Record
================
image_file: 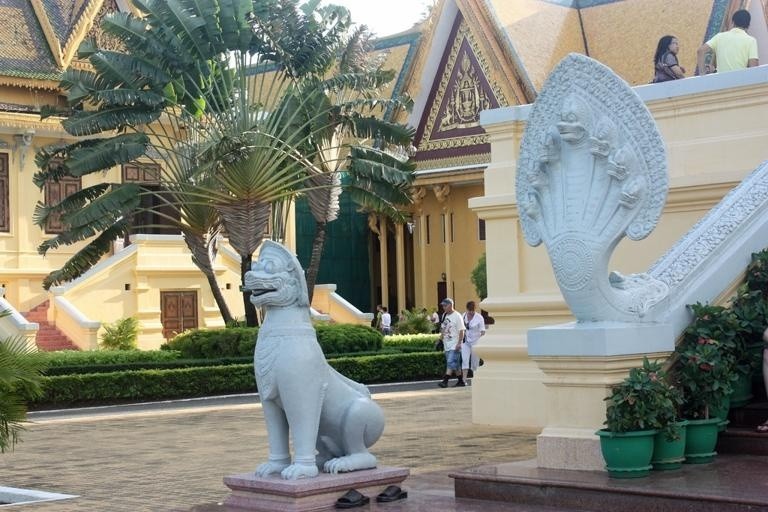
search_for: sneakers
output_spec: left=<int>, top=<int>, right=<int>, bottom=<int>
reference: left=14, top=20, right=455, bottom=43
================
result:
left=456, top=381, right=465, bottom=386
left=438, top=381, right=448, bottom=387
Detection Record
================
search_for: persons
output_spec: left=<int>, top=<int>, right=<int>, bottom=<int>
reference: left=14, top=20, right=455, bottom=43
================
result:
left=651, top=36, right=685, bottom=83
left=697, top=10, right=759, bottom=76
left=439, top=298, right=464, bottom=388
left=461, top=301, right=485, bottom=384
left=373, top=304, right=439, bottom=336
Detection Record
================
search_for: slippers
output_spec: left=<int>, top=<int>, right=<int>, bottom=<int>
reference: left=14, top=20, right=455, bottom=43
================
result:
left=376, top=485, right=407, bottom=501
left=336, top=488, right=370, bottom=508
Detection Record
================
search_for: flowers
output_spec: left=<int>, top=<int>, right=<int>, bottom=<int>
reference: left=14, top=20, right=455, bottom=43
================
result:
left=744, top=247, right=768, bottom=304
left=685, top=304, right=755, bottom=366
left=603, top=368, right=663, bottom=432
left=674, top=338, right=733, bottom=420
left=653, top=373, right=689, bottom=440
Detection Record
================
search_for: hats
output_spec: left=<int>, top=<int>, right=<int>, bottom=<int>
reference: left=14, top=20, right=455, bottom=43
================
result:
left=440, top=298, right=454, bottom=305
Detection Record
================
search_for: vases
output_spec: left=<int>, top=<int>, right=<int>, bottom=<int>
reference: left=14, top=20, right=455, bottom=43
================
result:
left=685, top=419, right=722, bottom=464
left=596, top=428, right=658, bottom=478
left=726, top=365, right=753, bottom=408
left=651, top=417, right=689, bottom=470
